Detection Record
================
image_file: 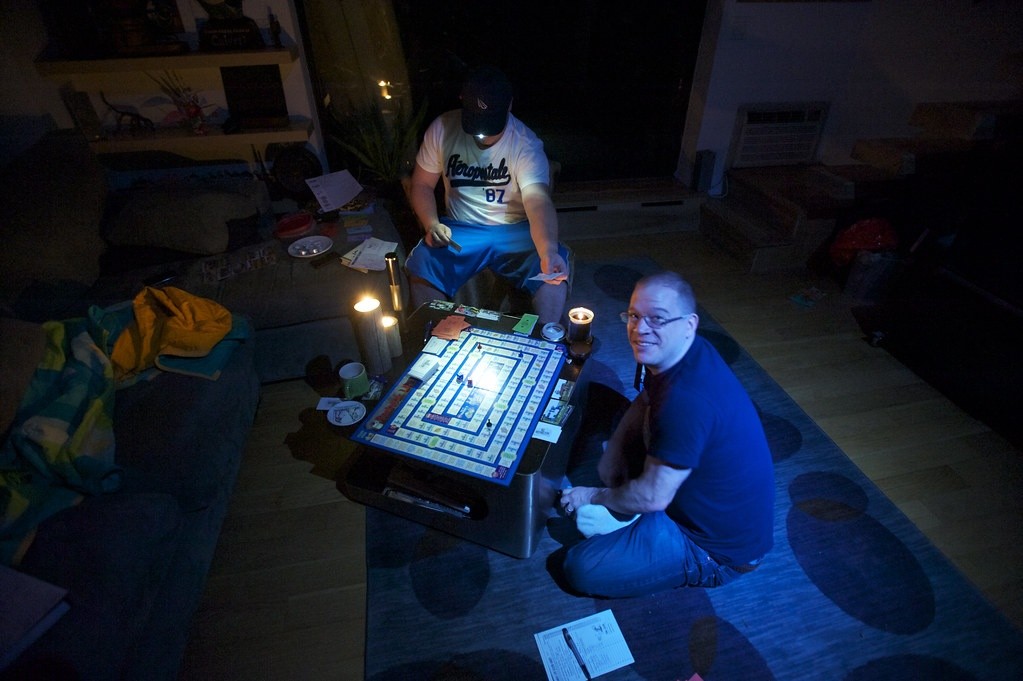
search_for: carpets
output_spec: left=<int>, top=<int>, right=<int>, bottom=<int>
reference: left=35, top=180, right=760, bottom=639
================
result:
left=361, top=254, right=1023, bottom=681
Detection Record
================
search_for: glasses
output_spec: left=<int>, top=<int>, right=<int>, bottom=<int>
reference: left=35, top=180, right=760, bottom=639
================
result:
left=619, top=312, right=689, bottom=329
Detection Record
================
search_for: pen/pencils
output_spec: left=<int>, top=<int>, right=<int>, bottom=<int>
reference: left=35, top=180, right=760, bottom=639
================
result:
left=562, top=628, right=592, bottom=681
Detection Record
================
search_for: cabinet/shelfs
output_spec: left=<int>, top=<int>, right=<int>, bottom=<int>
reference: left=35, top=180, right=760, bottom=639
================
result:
left=34, top=37, right=312, bottom=155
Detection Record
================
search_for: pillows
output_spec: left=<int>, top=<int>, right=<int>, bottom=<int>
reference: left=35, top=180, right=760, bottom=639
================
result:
left=0, top=131, right=105, bottom=288
left=0, top=318, right=47, bottom=436
left=99, top=190, right=229, bottom=255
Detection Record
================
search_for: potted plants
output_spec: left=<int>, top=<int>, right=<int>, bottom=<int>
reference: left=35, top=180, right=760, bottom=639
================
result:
left=322, top=102, right=432, bottom=240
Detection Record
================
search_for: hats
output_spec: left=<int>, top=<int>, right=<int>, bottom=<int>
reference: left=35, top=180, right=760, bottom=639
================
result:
left=462, top=65, right=512, bottom=136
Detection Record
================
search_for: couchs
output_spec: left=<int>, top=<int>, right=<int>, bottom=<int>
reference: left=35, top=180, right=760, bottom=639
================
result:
left=0, top=122, right=407, bottom=681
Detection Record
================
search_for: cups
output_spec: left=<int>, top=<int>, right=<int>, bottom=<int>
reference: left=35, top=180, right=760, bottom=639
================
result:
left=567, top=307, right=595, bottom=344
left=338, top=363, right=370, bottom=400
left=383, top=315, right=403, bottom=357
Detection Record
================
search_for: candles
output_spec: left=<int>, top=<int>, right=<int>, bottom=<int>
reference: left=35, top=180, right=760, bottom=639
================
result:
left=353, top=296, right=403, bottom=375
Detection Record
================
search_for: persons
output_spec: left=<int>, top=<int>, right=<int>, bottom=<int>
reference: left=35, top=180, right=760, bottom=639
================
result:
left=560, top=272, right=775, bottom=597
left=406, top=61, right=570, bottom=327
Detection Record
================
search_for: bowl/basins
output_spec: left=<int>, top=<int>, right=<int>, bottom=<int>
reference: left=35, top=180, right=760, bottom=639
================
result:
left=273, top=212, right=317, bottom=244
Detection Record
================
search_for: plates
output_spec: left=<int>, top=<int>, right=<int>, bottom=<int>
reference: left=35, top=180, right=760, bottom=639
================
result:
left=287, top=236, right=332, bottom=257
left=327, top=400, right=366, bottom=426
